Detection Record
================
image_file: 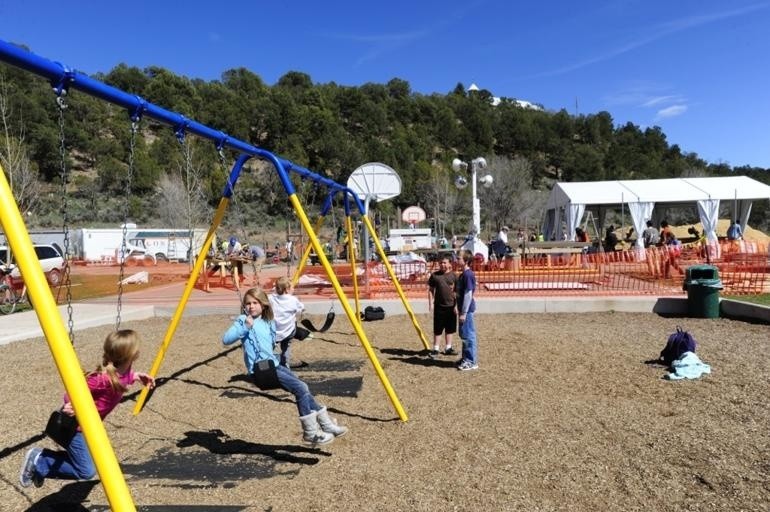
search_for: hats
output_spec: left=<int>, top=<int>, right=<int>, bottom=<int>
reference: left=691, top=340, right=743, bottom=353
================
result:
left=230, top=238, right=236, bottom=246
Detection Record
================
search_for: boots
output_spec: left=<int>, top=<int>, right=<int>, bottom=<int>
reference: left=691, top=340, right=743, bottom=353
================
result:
left=315, top=406, right=349, bottom=438
left=299, top=412, right=335, bottom=446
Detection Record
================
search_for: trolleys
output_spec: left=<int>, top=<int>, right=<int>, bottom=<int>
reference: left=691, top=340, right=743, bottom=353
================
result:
left=307, top=253, right=336, bottom=266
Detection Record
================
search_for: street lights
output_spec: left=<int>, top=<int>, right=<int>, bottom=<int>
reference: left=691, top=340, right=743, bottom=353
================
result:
left=450, top=152, right=495, bottom=263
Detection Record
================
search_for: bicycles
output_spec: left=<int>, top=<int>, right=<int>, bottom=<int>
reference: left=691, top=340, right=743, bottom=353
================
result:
left=0, top=267, right=33, bottom=314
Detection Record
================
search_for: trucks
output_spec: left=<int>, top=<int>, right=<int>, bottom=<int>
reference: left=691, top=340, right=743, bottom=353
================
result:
left=377, top=228, right=432, bottom=253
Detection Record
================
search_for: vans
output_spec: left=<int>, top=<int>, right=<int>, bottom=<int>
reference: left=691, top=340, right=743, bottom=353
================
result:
left=0, top=243, right=67, bottom=290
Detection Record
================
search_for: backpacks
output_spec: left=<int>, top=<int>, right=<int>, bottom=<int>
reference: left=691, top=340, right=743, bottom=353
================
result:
left=659, top=325, right=695, bottom=365
left=364, top=306, right=384, bottom=321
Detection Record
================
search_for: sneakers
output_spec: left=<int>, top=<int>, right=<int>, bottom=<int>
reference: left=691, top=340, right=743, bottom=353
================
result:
left=458, top=360, right=479, bottom=370
left=445, top=348, right=458, bottom=355
left=19, top=449, right=41, bottom=488
left=433, top=350, right=440, bottom=355
left=33, top=472, right=44, bottom=488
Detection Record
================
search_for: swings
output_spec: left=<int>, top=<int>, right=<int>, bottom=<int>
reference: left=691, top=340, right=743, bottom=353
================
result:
left=176, top=115, right=285, bottom=390
left=46, top=61, right=146, bottom=450
left=354, top=202, right=384, bottom=321
left=301, top=172, right=335, bottom=333
left=274, top=160, right=311, bottom=341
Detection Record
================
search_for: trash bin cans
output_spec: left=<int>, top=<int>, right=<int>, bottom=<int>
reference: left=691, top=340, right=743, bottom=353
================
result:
left=683, top=265, right=724, bottom=318
left=504, top=252, right=521, bottom=271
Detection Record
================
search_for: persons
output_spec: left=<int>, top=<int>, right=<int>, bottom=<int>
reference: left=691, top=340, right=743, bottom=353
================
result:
left=428, top=253, right=458, bottom=355
left=263, top=239, right=293, bottom=260
left=574, top=221, right=745, bottom=280
left=321, top=222, right=390, bottom=262
left=489, top=226, right=567, bottom=264
left=438, top=234, right=464, bottom=269
left=19, top=329, right=156, bottom=488
left=207, top=237, right=265, bottom=285
left=408, top=221, right=414, bottom=229
left=454, top=250, right=479, bottom=371
left=267, top=276, right=305, bottom=372
left=222, top=288, right=349, bottom=445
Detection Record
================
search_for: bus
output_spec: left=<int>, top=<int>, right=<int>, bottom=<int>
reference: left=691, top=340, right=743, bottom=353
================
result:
left=114, top=229, right=213, bottom=266
